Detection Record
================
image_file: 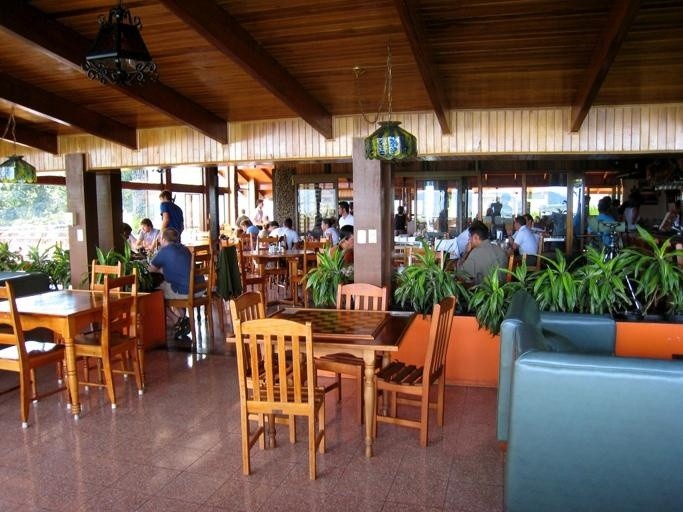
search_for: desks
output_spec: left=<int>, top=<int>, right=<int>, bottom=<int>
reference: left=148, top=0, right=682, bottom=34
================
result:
left=625, top=223, right=683, bottom=269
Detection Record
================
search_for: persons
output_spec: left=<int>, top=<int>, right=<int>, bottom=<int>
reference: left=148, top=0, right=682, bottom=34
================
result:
left=236, top=209, right=249, bottom=227
left=269, top=218, right=298, bottom=250
left=337, top=202, right=354, bottom=229
left=159, top=190, right=184, bottom=241
left=135, top=219, right=160, bottom=254
left=253, top=199, right=264, bottom=226
left=147, top=229, right=206, bottom=331
left=320, top=219, right=340, bottom=246
left=457, top=221, right=508, bottom=283
left=256, top=220, right=279, bottom=248
left=394, top=206, right=410, bottom=236
left=240, top=219, right=259, bottom=249
left=512, top=213, right=534, bottom=239
left=329, top=226, right=354, bottom=266
left=508, top=216, right=538, bottom=266
left=121, top=223, right=143, bottom=260
left=585, top=198, right=621, bottom=245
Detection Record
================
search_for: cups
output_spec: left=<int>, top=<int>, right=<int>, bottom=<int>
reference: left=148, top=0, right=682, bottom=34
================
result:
left=404, top=255, right=412, bottom=266
left=404, top=246, right=412, bottom=255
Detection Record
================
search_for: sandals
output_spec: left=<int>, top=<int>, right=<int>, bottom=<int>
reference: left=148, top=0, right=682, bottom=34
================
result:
left=174, top=317, right=189, bottom=340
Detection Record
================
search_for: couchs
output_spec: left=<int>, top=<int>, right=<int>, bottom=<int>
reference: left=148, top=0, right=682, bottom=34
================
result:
left=505, top=346, right=683, bottom=510
left=497, top=291, right=617, bottom=443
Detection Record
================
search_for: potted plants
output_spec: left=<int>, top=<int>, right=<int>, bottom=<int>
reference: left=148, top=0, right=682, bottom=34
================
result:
left=623, top=225, right=683, bottom=315
left=586, top=252, right=632, bottom=317
left=535, top=247, right=586, bottom=316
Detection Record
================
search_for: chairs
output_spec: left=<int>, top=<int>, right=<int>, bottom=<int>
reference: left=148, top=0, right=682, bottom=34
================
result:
left=522, top=234, right=544, bottom=273
left=197, top=245, right=235, bottom=330
left=163, top=247, right=213, bottom=351
left=308, top=283, right=388, bottom=426
left=224, top=241, right=265, bottom=308
left=0, top=281, right=67, bottom=427
left=409, top=246, right=443, bottom=275
left=63, top=265, right=144, bottom=409
left=504, top=254, right=515, bottom=284
left=368, top=298, right=456, bottom=446
left=56, top=260, right=127, bottom=392
left=230, top=291, right=319, bottom=444
left=233, top=319, right=324, bottom=480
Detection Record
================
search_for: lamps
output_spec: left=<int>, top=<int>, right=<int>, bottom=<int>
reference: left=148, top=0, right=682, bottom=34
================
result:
left=80, top=0, right=160, bottom=91
left=0, top=112, right=38, bottom=184
left=353, top=39, right=419, bottom=164
